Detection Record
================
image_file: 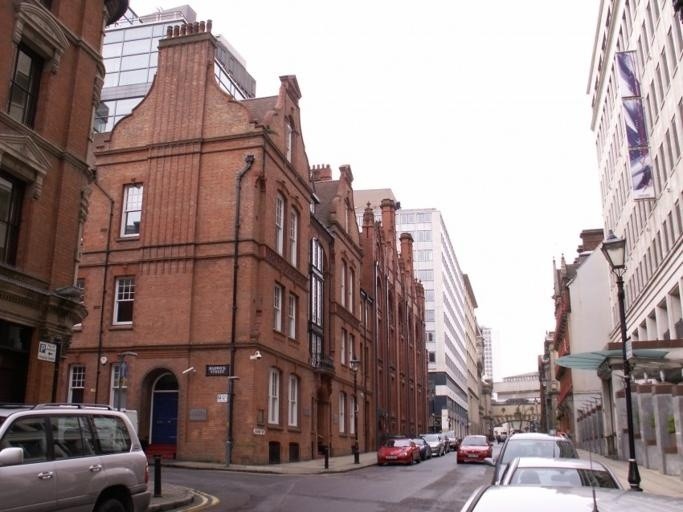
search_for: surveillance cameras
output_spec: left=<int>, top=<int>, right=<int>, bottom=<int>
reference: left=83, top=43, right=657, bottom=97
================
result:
left=256, top=354, right=262, bottom=360
left=181, top=366, right=194, bottom=375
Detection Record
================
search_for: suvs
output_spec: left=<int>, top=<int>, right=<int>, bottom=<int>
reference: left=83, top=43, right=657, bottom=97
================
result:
left=0, top=403, right=150, bottom=512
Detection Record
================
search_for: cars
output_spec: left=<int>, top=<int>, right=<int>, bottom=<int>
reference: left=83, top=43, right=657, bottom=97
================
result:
left=457, top=430, right=682, bottom=511
left=376, top=430, right=459, bottom=465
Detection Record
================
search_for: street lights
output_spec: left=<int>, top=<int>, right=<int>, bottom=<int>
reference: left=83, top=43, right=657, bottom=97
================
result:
left=117, top=351, right=137, bottom=410
left=601, top=230, right=641, bottom=490
left=431, top=390, right=434, bottom=434
left=350, top=357, right=360, bottom=463
